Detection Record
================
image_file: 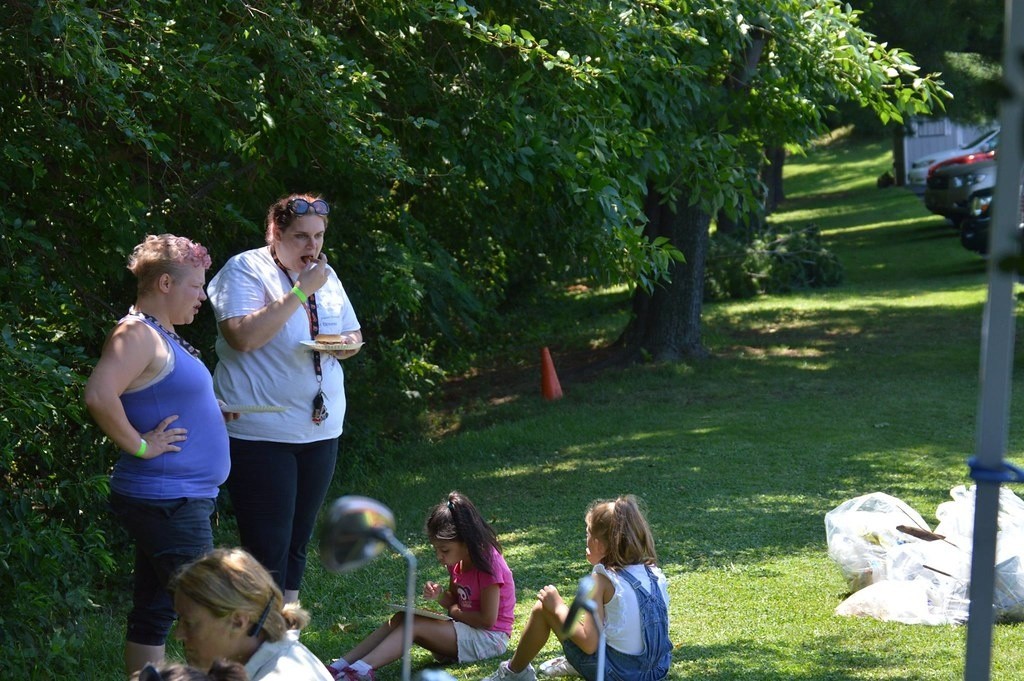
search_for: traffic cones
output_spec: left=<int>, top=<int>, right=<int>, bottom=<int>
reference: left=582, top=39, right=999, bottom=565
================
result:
left=536, top=346, right=564, bottom=402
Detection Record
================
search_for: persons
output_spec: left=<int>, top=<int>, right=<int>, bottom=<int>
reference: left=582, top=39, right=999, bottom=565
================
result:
left=85, top=233, right=231, bottom=680
left=205, top=191, right=364, bottom=602
left=172, top=548, right=336, bottom=681
left=327, top=492, right=517, bottom=680
left=128, top=658, right=250, bottom=681
left=481, top=494, right=674, bottom=680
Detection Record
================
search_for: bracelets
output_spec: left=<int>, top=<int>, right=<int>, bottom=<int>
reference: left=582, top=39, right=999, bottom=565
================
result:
left=434, top=589, right=444, bottom=602
left=290, top=285, right=307, bottom=303
left=134, top=439, right=147, bottom=458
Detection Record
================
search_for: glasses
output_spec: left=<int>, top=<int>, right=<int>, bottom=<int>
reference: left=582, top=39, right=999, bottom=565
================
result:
left=277, top=198, right=330, bottom=219
left=137, top=661, right=162, bottom=681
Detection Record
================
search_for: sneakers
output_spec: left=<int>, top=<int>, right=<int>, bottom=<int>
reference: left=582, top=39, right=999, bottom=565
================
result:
left=483, top=660, right=538, bottom=681
left=327, top=660, right=375, bottom=681
left=540, top=655, right=580, bottom=675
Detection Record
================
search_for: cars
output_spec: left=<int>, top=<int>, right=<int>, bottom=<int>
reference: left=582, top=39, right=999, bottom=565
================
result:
left=909, top=124, right=1023, bottom=267
